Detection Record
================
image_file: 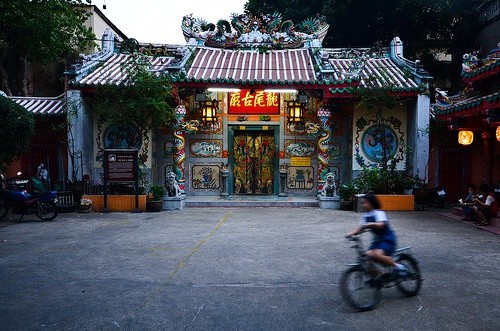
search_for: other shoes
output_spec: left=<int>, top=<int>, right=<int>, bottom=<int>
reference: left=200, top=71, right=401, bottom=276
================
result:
left=461, top=217, right=473, bottom=221
left=394, top=264, right=408, bottom=275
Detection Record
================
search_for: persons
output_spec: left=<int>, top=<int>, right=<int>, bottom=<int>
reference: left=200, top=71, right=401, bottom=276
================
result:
left=458, top=182, right=498, bottom=226
left=344, top=190, right=409, bottom=284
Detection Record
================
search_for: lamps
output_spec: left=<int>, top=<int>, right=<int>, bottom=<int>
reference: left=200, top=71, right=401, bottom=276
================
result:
left=298, top=91, right=308, bottom=103
left=195, top=90, right=205, bottom=101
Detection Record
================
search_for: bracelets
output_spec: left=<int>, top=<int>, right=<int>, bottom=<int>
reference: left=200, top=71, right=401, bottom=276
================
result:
left=465, top=202, right=467, bottom=205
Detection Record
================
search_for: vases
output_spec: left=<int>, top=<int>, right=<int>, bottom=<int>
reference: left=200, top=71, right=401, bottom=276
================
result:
left=373, top=192, right=414, bottom=211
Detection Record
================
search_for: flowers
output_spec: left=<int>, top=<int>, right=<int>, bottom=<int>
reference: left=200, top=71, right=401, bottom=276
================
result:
left=349, top=165, right=432, bottom=195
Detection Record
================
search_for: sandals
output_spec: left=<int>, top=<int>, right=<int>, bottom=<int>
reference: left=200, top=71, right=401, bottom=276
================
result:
left=473, top=220, right=482, bottom=224
left=477, top=222, right=488, bottom=226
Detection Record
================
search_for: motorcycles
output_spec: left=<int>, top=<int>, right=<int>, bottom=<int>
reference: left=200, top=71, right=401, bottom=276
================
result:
left=0, top=174, right=60, bottom=223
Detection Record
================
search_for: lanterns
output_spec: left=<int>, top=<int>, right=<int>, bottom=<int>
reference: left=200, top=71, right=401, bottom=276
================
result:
left=457, top=131, right=474, bottom=146
left=496, top=126, right=500, bottom=141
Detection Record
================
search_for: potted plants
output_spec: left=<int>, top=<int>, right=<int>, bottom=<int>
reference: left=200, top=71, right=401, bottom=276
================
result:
left=338, top=178, right=356, bottom=211
left=83, top=183, right=149, bottom=212
left=146, top=184, right=165, bottom=212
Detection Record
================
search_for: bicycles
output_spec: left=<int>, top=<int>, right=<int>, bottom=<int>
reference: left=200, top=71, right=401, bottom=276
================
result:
left=339, top=228, right=421, bottom=312
